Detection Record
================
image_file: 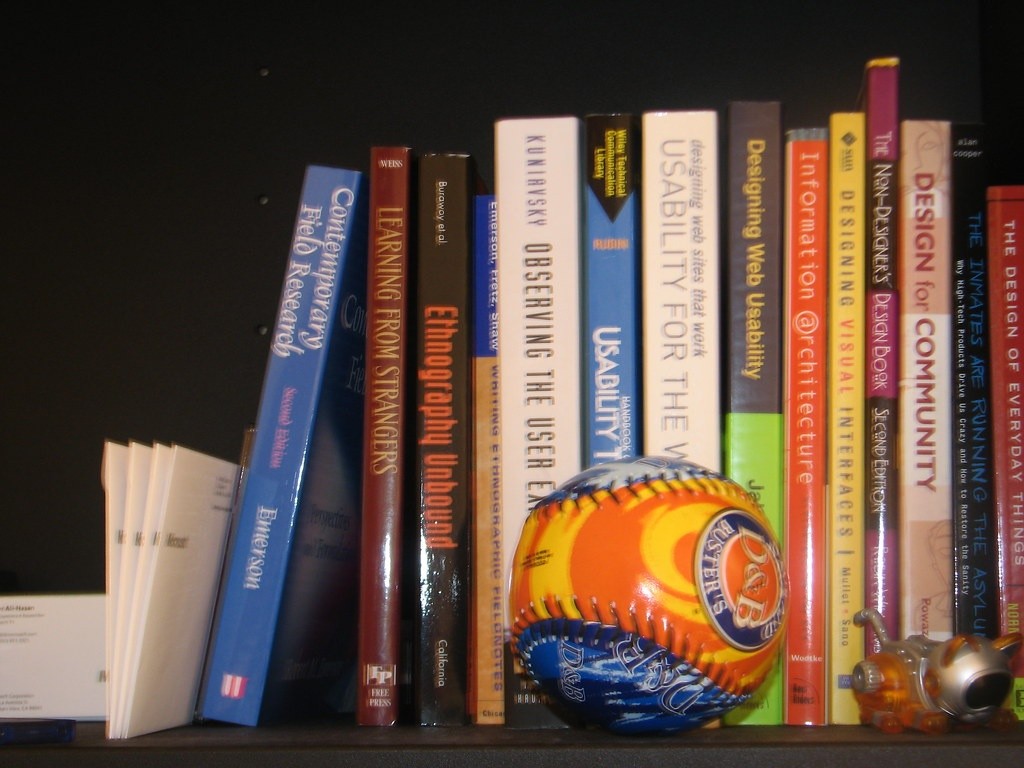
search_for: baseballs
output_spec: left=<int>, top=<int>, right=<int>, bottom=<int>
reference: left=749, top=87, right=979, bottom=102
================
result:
left=508, top=455, right=792, bottom=740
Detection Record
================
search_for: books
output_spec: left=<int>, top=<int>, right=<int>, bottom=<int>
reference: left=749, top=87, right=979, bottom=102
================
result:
left=102, top=440, right=236, bottom=741
left=359, top=57, right=1023, bottom=728
left=197, top=167, right=367, bottom=729
